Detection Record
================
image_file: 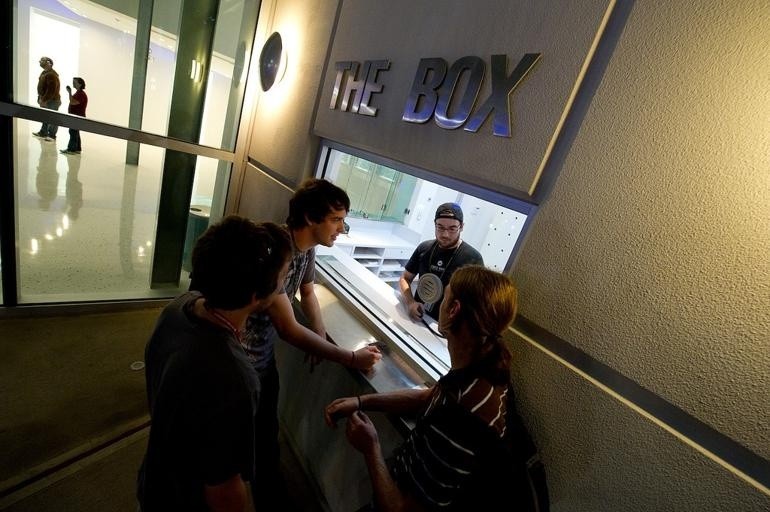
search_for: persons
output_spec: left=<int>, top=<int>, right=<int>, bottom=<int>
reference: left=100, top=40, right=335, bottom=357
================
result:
left=136, top=217, right=292, bottom=510
left=35, top=137, right=59, bottom=211
left=32, top=56, right=61, bottom=140
left=60, top=77, right=88, bottom=155
left=237, top=177, right=385, bottom=511
left=65, top=154, right=83, bottom=220
left=400, top=204, right=483, bottom=324
left=326, top=263, right=518, bottom=510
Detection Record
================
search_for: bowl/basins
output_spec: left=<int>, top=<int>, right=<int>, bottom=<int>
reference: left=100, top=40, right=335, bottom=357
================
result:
left=212, top=313, right=244, bottom=343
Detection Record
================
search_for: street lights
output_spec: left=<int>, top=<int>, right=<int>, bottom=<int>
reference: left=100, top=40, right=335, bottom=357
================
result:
left=453, top=412, right=551, bottom=511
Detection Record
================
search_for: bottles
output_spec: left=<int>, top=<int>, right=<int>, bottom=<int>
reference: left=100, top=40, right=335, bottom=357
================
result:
left=434, top=202, right=464, bottom=222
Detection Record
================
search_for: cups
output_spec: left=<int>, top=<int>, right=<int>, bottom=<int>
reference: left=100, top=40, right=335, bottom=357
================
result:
left=242, top=222, right=274, bottom=282
left=435, top=223, right=461, bottom=233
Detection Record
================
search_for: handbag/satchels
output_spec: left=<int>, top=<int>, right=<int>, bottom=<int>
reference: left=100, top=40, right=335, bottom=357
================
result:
left=349, top=349, right=357, bottom=368
left=357, top=394, right=363, bottom=416
